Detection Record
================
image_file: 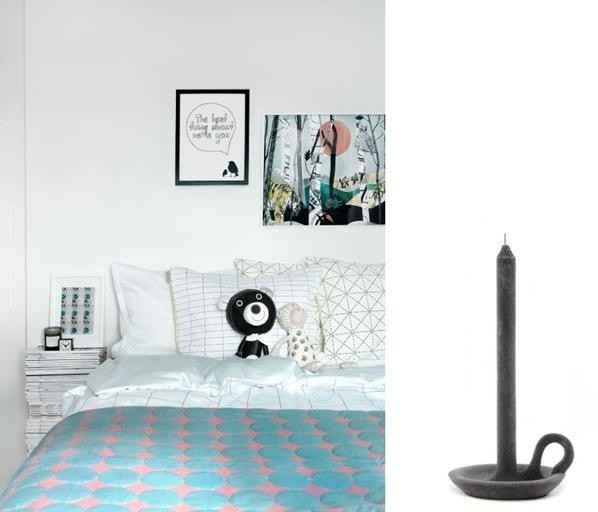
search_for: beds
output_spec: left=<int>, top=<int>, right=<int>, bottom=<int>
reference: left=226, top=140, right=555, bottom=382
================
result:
left=0, top=354, right=385, bottom=512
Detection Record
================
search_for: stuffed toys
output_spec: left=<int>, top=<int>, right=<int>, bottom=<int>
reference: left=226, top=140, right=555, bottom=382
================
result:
left=271, top=302, right=357, bottom=373
left=217, top=286, right=276, bottom=360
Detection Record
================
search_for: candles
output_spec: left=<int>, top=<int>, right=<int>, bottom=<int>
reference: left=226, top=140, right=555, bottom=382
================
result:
left=495, top=232, right=516, bottom=479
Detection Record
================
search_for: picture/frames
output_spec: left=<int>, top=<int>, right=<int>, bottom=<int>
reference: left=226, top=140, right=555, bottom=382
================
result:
left=175, top=89, right=249, bottom=185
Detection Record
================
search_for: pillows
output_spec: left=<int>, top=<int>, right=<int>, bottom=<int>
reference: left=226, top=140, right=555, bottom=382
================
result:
left=111, top=256, right=385, bottom=364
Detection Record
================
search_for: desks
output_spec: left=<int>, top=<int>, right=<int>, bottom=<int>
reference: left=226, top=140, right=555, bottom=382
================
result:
left=24, top=347, right=107, bottom=457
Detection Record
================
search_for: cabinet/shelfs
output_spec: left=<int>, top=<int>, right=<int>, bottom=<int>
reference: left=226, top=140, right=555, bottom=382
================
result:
left=48, top=273, right=106, bottom=349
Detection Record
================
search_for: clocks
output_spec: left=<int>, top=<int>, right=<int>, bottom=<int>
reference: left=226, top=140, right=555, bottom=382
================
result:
left=58, top=339, right=72, bottom=351
